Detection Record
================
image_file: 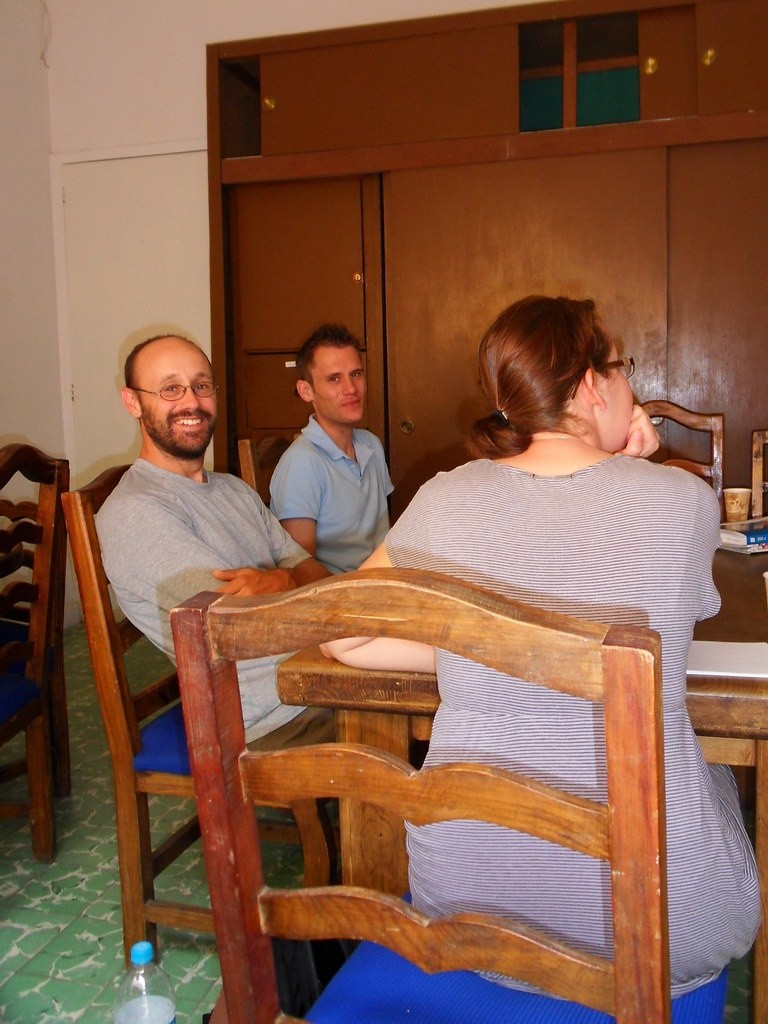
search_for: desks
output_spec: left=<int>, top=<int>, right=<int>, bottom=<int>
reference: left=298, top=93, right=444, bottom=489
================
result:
left=278, top=545, right=768, bottom=1024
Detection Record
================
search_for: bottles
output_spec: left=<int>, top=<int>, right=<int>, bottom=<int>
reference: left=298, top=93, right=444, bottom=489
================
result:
left=115, top=941, right=176, bottom=1024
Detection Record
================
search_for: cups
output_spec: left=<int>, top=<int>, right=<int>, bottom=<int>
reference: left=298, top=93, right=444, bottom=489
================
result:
left=763, top=571, right=768, bottom=609
left=723, top=488, right=752, bottom=523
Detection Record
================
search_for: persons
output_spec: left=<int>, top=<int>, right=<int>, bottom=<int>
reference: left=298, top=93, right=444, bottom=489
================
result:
left=325, top=295, right=761, bottom=1002
left=268, top=319, right=395, bottom=573
left=95, top=334, right=430, bottom=814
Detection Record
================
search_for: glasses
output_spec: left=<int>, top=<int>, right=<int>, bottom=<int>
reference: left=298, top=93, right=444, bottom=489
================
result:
left=132, top=380, right=219, bottom=401
left=572, top=354, right=636, bottom=399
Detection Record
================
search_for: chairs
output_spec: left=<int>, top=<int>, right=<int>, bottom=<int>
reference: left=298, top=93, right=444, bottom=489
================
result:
left=639, top=399, right=768, bottom=530
left=0, top=432, right=336, bottom=969
left=169, top=568, right=671, bottom=1024
